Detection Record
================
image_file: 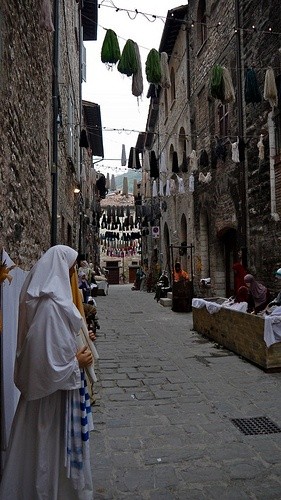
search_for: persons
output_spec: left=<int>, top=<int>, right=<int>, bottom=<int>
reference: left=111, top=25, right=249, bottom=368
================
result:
left=265, top=267, right=281, bottom=314
left=134, top=268, right=147, bottom=290
left=237, top=274, right=267, bottom=314
left=2, top=245, right=99, bottom=500
left=79, top=259, right=101, bottom=332
left=172, top=262, right=190, bottom=283
left=231, top=263, right=247, bottom=302
left=154, top=270, right=169, bottom=303
